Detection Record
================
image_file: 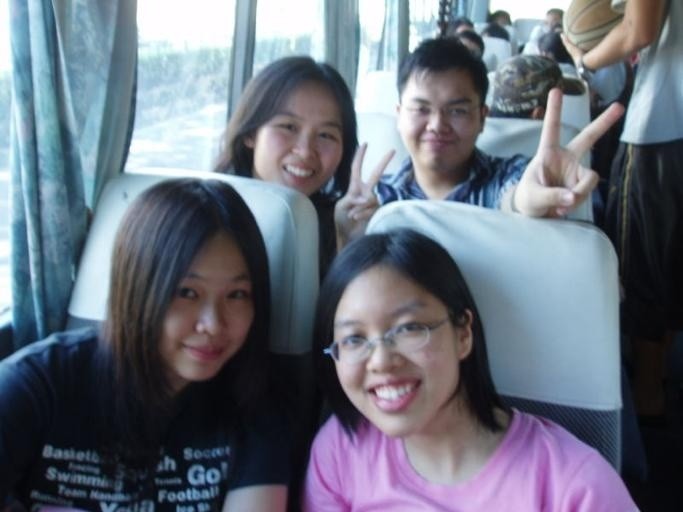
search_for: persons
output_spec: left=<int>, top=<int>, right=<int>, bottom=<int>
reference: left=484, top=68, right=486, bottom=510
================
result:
left=369, top=10, right=632, bottom=219
left=0, top=176, right=292, bottom=512
left=557, top=0, right=683, bottom=425
left=210, top=54, right=397, bottom=274
left=297, top=231, right=643, bottom=512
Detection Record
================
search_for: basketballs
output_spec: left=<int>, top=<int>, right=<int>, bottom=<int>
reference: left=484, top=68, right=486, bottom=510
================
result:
left=563, top=0, right=625, bottom=55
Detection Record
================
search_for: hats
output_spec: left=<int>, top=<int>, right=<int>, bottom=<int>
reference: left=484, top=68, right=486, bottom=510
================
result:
left=493, top=55, right=585, bottom=113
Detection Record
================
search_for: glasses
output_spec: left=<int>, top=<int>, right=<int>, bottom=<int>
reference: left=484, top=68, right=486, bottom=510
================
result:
left=323, top=317, right=452, bottom=365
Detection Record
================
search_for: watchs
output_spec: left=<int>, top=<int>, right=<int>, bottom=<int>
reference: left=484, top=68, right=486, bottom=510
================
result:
left=575, top=57, right=597, bottom=82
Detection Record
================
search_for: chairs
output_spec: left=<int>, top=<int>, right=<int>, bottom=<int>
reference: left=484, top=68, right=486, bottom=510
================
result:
left=354, top=68, right=402, bottom=111
left=351, top=108, right=409, bottom=185
left=487, top=69, right=593, bottom=129
left=473, top=113, right=597, bottom=225
left=361, top=199, right=622, bottom=512
left=466, top=19, right=578, bottom=73
left=62, top=167, right=319, bottom=512
